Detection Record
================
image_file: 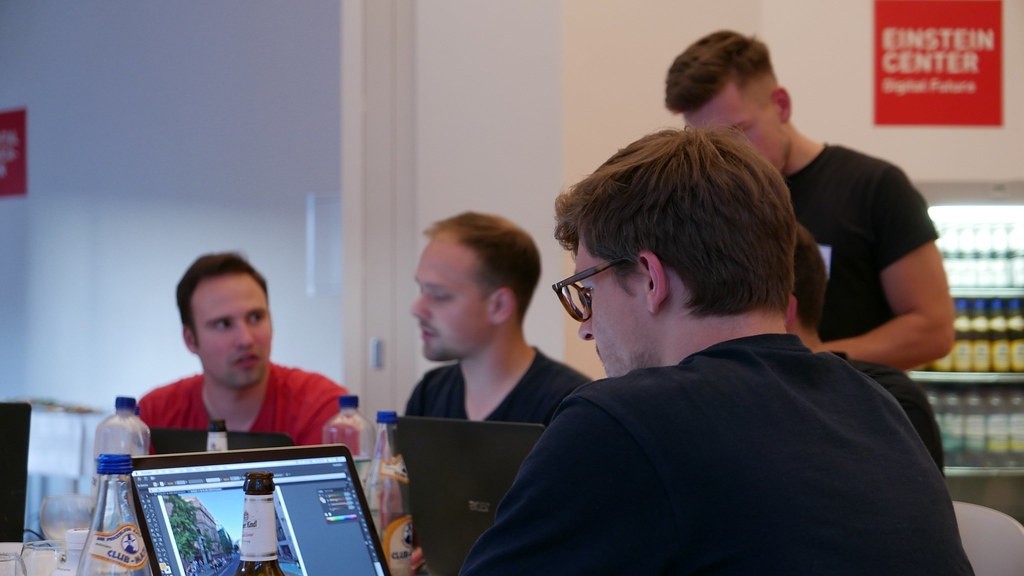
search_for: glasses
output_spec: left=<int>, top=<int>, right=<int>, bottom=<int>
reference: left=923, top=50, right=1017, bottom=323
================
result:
left=552, top=258, right=638, bottom=322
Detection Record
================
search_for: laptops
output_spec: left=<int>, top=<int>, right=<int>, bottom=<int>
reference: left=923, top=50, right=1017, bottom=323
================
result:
left=130, top=428, right=392, bottom=576
left=391, top=415, right=546, bottom=576
left=0, top=402, right=32, bottom=543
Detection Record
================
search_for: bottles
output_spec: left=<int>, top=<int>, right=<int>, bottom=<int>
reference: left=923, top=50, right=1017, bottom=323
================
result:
left=233, top=471, right=287, bottom=576
left=935, top=297, right=1024, bottom=371
left=207, top=419, right=228, bottom=451
left=134, top=407, right=150, bottom=455
left=935, top=226, right=1024, bottom=288
left=89, top=397, right=144, bottom=518
left=324, top=396, right=375, bottom=486
left=77, top=453, right=154, bottom=576
left=927, top=393, right=1024, bottom=466
left=365, top=410, right=415, bottom=576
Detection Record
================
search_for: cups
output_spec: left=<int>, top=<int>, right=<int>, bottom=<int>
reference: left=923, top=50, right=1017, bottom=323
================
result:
left=0, top=553, right=21, bottom=576
left=21, top=541, right=67, bottom=576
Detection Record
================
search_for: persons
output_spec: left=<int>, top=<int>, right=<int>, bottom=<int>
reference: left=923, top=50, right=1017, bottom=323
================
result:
left=135, top=252, right=360, bottom=456
left=404, top=212, right=592, bottom=424
left=457, top=30, right=974, bottom=576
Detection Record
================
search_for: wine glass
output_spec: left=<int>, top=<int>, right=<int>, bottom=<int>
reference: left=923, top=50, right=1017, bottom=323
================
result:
left=41, top=495, right=95, bottom=557
